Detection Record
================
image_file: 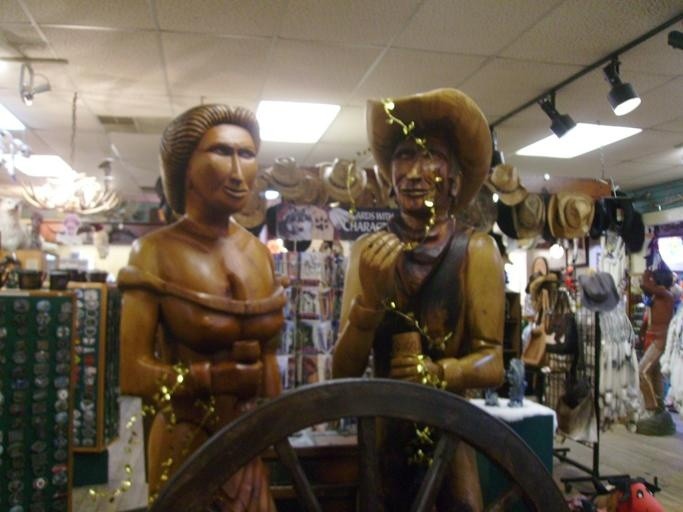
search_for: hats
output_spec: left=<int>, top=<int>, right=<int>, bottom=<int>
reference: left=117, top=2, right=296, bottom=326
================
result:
left=483, top=165, right=596, bottom=240
left=264, top=156, right=368, bottom=208
left=234, top=178, right=267, bottom=229
left=581, top=272, right=619, bottom=312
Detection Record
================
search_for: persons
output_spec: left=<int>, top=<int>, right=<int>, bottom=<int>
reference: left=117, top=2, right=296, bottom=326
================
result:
left=119, top=103, right=288, bottom=510
left=634, top=269, right=675, bottom=413
left=331, top=86, right=507, bottom=512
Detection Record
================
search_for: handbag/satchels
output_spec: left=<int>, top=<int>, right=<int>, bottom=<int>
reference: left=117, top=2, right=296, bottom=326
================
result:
left=521, top=260, right=577, bottom=367
left=563, top=376, right=589, bottom=408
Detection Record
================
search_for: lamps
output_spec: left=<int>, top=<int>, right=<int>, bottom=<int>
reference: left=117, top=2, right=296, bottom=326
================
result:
left=487, top=15, right=683, bottom=168
